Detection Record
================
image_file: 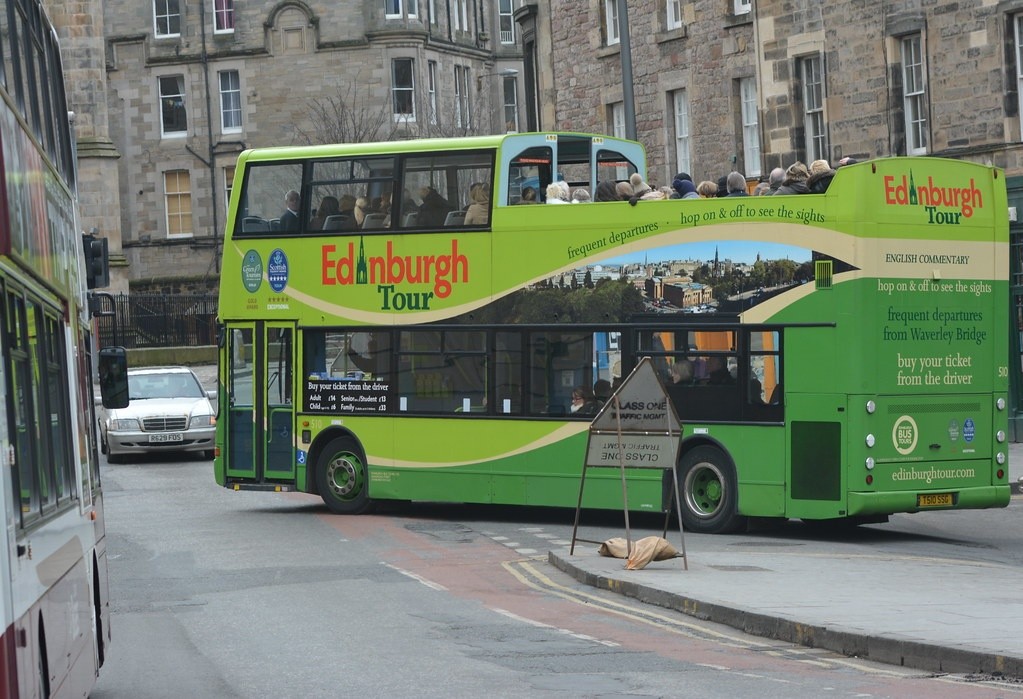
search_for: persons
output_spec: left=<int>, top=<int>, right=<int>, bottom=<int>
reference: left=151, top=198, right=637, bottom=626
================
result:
left=696, top=179, right=719, bottom=198
left=517, top=186, right=541, bottom=204
left=545, top=179, right=572, bottom=204
left=310, top=187, right=454, bottom=232
left=568, top=385, right=596, bottom=416
left=592, top=378, right=622, bottom=405
left=280, top=190, right=301, bottom=231
left=461, top=181, right=489, bottom=224
left=669, top=172, right=702, bottom=198
left=715, top=176, right=730, bottom=197
left=771, top=161, right=810, bottom=195
left=346, top=340, right=376, bottom=380
left=724, top=170, right=749, bottom=197
left=836, top=156, right=856, bottom=170
left=805, top=159, right=837, bottom=194
left=754, top=167, right=786, bottom=196
left=594, top=173, right=673, bottom=206
left=657, top=344, right=765, bottom=404
left=570, top=188, right=591, bottom=202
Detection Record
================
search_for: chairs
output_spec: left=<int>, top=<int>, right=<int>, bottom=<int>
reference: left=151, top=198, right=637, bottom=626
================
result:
left=244, top=209, right=466, bottom=232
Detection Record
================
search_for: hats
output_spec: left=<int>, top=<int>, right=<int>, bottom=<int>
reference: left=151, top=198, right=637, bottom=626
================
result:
left=673, top=179, right=695, bottom=196
left=630, top=173, right=652, bottom=198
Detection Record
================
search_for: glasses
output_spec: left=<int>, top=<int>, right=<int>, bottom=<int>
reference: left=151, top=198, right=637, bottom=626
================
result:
left=571, top=396, right=583, bottom=402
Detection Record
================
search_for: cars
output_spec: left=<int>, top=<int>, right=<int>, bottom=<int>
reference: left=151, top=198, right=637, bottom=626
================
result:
left=95, top=365, right=219, bottom=463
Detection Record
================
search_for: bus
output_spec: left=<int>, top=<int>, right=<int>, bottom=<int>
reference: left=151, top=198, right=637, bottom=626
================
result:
left=209, top=127, right=1014, bottom=533
left=0, top=0, right=134, bottom=699
